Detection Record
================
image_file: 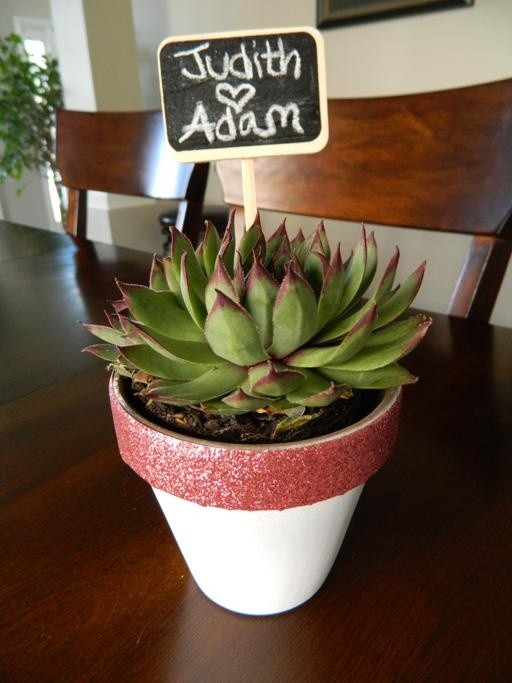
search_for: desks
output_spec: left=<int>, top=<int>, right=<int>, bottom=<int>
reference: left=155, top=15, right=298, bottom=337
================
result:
left=0, top=219, right=512, bottom=683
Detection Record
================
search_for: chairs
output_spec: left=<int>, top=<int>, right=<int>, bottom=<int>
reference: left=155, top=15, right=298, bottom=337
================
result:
left=215, top=77, right=512, bottom=323
left=56, top=108, right=209, bottom=251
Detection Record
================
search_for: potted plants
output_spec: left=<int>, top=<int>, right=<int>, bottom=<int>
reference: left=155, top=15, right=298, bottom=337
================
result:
left=76, top=205, right=435, bottom=617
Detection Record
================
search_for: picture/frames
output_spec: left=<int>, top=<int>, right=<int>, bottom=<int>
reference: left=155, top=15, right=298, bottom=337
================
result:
left=316, top=0, right=474, bottom=29
